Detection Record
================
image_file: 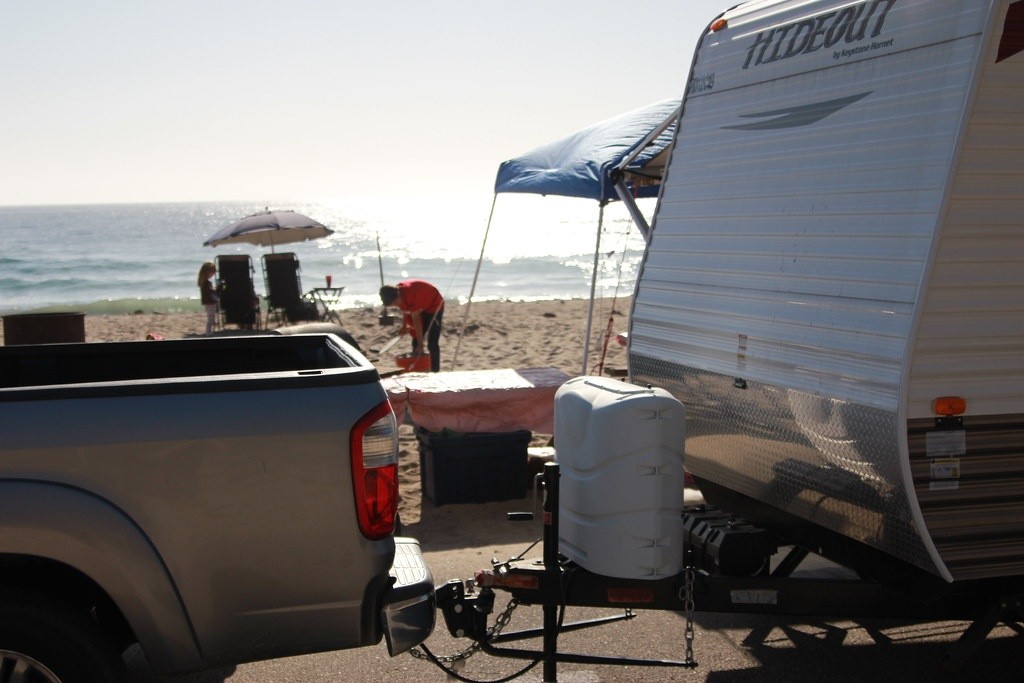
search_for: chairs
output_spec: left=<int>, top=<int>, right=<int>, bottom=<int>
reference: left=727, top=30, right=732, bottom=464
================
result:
left=214, top=255, right=262, bottom=333
left=261, top=252, right=318, bottom=330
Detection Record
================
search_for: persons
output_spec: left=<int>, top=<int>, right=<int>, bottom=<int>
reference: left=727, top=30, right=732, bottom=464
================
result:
left=379, top=279, right=445, bottom=372
left=198, top=262, right=219, bottom=337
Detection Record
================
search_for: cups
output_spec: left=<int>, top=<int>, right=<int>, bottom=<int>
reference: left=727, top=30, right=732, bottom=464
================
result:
left=327, top=276, right=332, bottom=288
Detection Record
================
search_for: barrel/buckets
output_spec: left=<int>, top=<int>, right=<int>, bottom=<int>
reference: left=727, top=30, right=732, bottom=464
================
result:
left=395, top=352, right=432, bottom=372
left=553, top=371, right=687, bottom=580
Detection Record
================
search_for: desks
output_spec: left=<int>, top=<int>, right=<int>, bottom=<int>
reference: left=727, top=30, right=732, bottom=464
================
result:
left=313, top=287, right=345, bottom=326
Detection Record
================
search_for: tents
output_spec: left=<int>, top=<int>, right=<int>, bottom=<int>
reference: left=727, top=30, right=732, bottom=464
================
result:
left=452, top=98, right=686, bottom=378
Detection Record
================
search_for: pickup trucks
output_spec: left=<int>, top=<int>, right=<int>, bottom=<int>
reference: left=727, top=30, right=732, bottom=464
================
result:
left=0, top=330, right=439, bottom=683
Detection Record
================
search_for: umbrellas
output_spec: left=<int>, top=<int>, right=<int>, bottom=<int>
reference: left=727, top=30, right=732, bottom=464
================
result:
left=202, top=206, right=336, bottom=255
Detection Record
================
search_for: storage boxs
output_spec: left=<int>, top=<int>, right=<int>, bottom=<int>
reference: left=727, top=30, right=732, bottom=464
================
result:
left=414, top=426, right=533, bottom=507
